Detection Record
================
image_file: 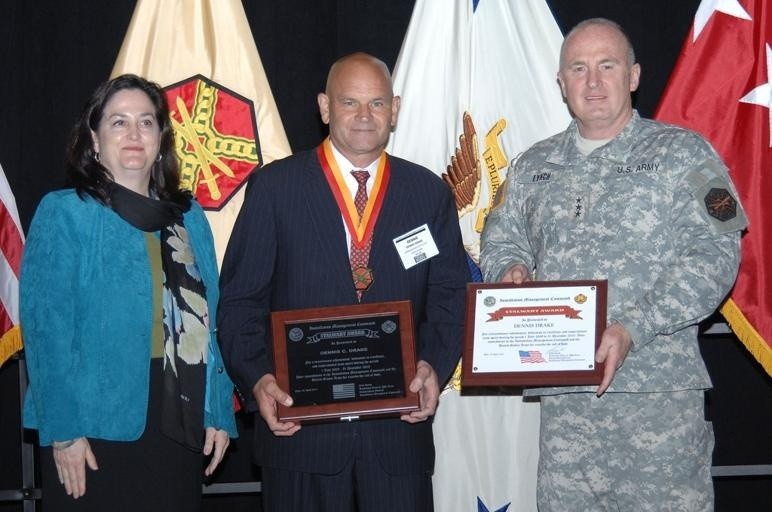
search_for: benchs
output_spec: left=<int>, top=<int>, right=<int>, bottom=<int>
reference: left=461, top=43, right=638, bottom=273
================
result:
left=1, top=161, right=26, bottom=375
left=380, top=2, right=576, bottom=512
left=651, top=0, right=772, bottom=384
left=105, top=0, right=296, bottom=278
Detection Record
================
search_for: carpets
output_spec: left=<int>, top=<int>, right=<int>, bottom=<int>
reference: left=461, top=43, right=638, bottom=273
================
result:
left=344, top=168, right=375, bottom=306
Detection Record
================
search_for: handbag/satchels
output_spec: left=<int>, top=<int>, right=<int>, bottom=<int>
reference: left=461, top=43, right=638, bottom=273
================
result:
left=52, top=439, right=82, bottom=450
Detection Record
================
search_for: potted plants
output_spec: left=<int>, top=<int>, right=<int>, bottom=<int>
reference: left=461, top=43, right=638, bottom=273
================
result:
left=270, top=301, right=423, bottom=424
left=460, top=279, right=608, bottom=388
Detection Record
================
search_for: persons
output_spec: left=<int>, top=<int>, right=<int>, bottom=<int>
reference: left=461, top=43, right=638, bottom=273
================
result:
left=12, top=73, right=240, bottom=511
left=474, top=15, right=749, bottom=511
left=215, top=48, right=476, bottom=511
